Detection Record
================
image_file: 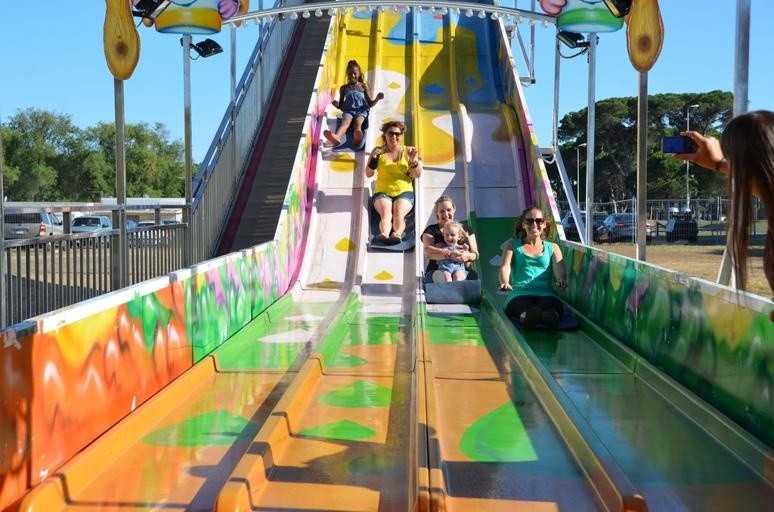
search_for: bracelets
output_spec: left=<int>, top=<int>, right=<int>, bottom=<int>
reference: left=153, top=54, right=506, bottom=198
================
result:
left=409, top=160, right=418, bottom=169
left=715, top=157, right=725, bottom=175
left=367, top=156, right=378, bottom=170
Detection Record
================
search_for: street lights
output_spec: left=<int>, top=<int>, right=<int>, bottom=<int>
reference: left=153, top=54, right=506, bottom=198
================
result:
left=576, top=143, right=586, bottom=209
left=686, top=104, right=698, bottom=208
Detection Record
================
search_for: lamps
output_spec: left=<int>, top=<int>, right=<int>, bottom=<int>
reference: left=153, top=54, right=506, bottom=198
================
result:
left=603, top=0, right=633, bottom=18
left=180, top=38, right=223, bottom=58
left=132, top=0, right=172, bottom=20
left=556, top=31, right=599, bottom=49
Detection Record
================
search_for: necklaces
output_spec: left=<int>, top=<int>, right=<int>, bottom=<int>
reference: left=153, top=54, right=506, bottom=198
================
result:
left=348, top=81, right=358, bottom=90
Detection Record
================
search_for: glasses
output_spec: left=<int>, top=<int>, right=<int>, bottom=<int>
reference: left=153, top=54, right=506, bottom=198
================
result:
left=388, top=131, right=400, bottom=138
left=522, top=218, right=545, bottom=225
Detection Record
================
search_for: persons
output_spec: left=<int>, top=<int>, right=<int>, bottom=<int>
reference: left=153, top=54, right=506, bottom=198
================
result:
left=434, top=221, right=470, bottom=283
left=364, top=121, right=420, bottom=245
left=672, top=110, right=773, bottom=323
left=417, top=195, right=480, bottom=293
left=324, top=60, right=384, bottom=144
left=498, top=207, right=569, bottom=331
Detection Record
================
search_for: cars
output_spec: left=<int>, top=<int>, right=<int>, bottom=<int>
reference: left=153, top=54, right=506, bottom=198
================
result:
left=69, top=216, right=111, bottom=248
left=134, top=221, right=164, bottom=247
left=3, top=213, right=67, bottom=252
left=161, top=219, right=180, bottom=244
left=593, top=212, right=652, bottom=245
left=125, top=220, right=136, bottom=247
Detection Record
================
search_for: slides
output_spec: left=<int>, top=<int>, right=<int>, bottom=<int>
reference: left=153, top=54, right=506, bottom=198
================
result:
left=0, top=0, right=774, bottom=512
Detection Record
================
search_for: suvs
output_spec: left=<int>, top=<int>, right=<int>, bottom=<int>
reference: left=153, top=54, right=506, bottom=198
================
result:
left=561, top=211, right=586, bottom=240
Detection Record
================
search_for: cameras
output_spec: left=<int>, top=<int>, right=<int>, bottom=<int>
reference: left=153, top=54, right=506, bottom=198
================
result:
left=663, top=137, right=694, bottom=153
